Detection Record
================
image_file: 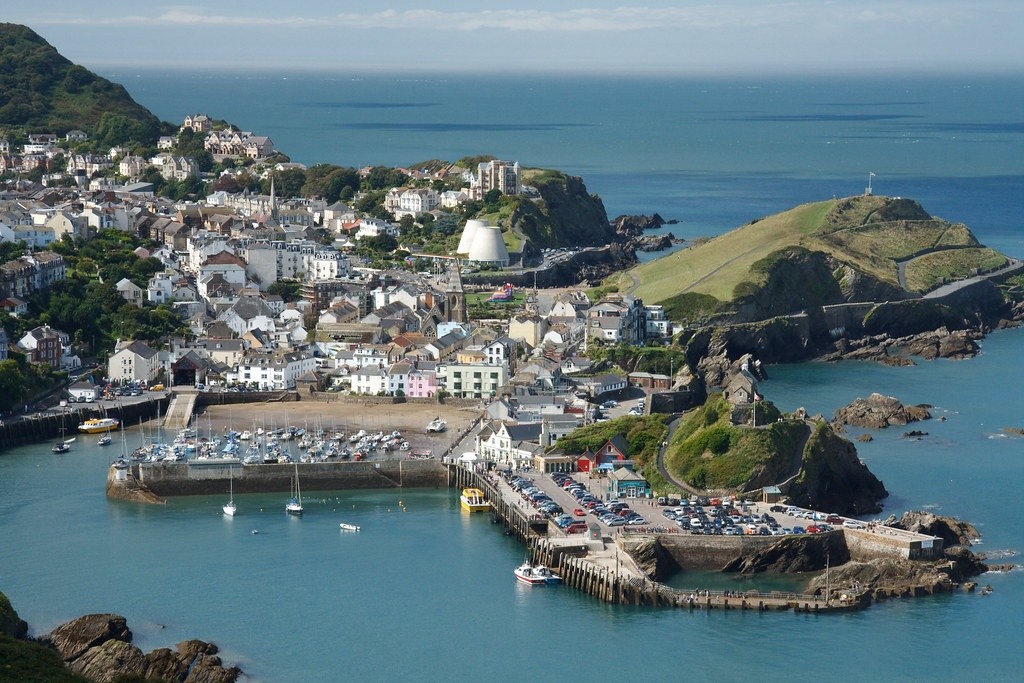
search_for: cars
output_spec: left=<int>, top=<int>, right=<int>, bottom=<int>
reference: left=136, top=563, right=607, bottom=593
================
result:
left=601, top=397, right=648, bottom=419
left=503, top=469, right=903, bottom=537
left=180, top=265, right=193, bottom=275
left=23, top=360, right=265, bottom=414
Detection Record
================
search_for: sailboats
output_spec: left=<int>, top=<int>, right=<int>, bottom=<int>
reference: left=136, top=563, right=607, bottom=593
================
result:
left=112, top=401, right=448, bottom=469
left=285, top=461, right=303, bottom=512
left=222, top=464, right=237, bottom=517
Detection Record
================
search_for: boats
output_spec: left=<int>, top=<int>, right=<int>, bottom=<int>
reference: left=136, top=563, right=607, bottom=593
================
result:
left=459, top=488, right=491, bottom=512
left=77, top=417, right=119, bottom=434
left=513, top=557, right=563, bottom=585
left=52, top=440, right=70, bottom=452
left=64, top=437, right=76, bottom=443
left=97, top=435, right=111, bottom=445
